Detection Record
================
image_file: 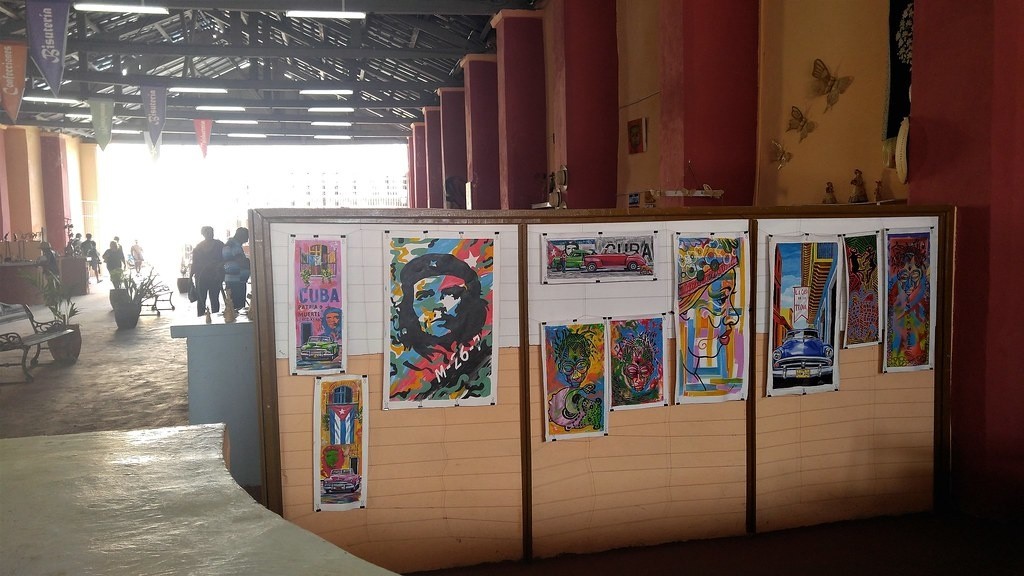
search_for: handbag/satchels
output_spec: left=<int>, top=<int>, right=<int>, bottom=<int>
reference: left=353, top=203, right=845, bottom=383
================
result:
left=188, top=283, right=198, bottom=303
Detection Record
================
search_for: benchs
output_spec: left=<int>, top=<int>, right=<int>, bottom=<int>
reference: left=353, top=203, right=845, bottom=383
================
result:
left=0, top=303, right=75, bottom=380
left=137, top=278, right=175, bottom=317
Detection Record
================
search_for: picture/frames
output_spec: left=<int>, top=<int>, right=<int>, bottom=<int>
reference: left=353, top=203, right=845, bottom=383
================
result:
left=466, top=182, right=471, bottom=210
left=627, top=116, right=646, bottom=155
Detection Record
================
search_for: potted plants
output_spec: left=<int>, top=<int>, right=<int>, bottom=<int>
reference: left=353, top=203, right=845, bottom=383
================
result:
left=111, top=267, right=163, bottom=329
left=177, top=266, right=191, bottom=293
left=109, top=267, right=129, bottom=310
left=42, top=270, right=82, bottom=364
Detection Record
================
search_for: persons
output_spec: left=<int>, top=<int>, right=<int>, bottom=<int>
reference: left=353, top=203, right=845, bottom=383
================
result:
left=848, top=168, right=869, bottom=203
left=130, top=239, right=143, bottom=277
left=112, top=235, right=124, bottom=249
left=821, top=181, right=838, bottom=204
left=36, top=240, right=62, bottom=307
left=205, top=307, right=211, bottom=320
left=185, top=224, right=227, bottom=315
left=71, top=232, right=84, bottom=252
left=872, top=180, right=883, bottom=202
left=225, top=288, right=236, bottom=314
left=223, top=298, right=235, bottom=323
left=102, top=239, right=127, bottom=287
left=219, top=227, right=251, bottom=313
left=79, top=232, right=104, bottom=284
